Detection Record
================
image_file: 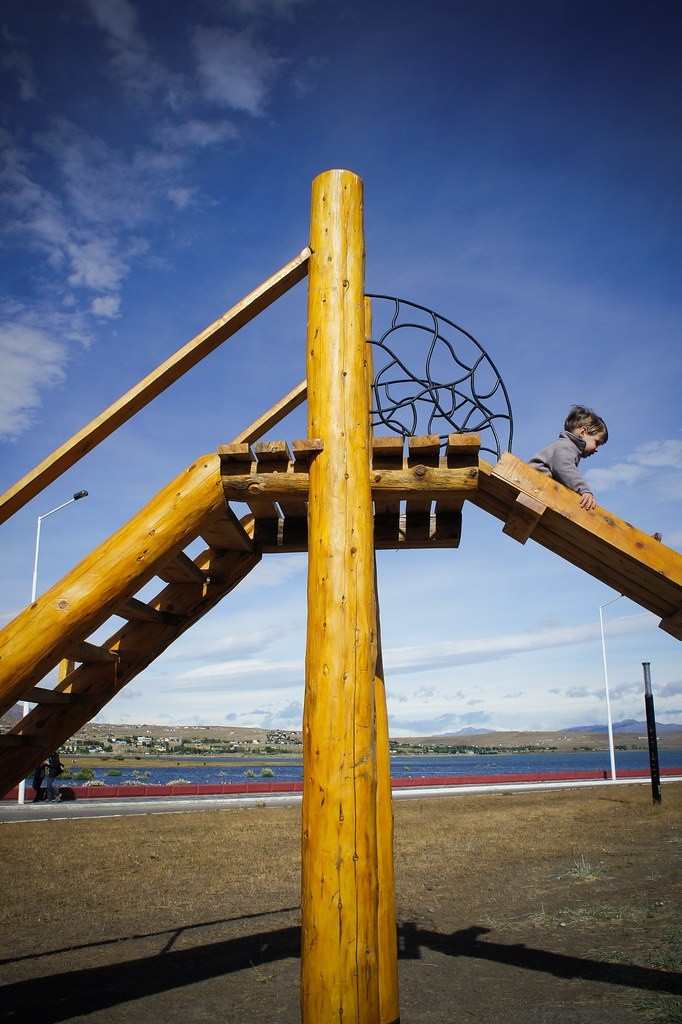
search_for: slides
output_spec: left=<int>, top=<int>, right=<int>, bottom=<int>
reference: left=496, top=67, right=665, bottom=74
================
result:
left=472, top=454, right=682, bottom=641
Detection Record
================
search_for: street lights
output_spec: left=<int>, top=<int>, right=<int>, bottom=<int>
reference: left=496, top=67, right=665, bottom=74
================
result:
left=18, top=490, right=89, bottom=805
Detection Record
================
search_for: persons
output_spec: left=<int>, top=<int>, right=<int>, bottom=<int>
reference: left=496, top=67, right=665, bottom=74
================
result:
left=43, top=751, right=65, bottom=803
left=522, top=403, right=663, bottom=544
left=31, top=760, right=47, bottom=804
